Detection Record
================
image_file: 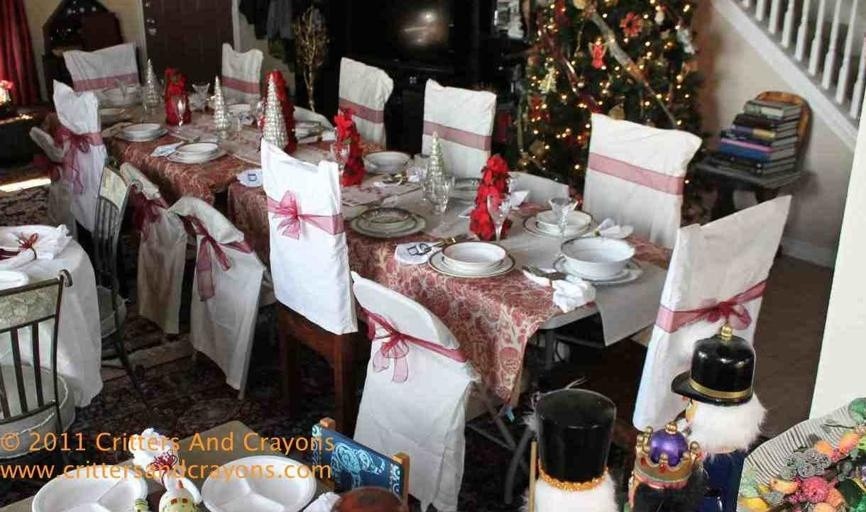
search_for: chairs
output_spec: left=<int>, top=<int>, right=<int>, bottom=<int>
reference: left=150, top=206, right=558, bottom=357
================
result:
left=0, top=41, right=792, bottom=512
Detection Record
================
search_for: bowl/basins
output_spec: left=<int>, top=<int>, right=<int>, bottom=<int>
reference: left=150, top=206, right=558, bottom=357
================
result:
left=123, top=123, right=159, bottom=133
left=362, top=206, right=414, bottom=228
left=175, top=143, right=219, bottom=155
left=103, top=86, right=140, bottom=104
left=98, top=108, right=124, bottom=117
left=536, top=208, right=590, bottom=228
left=558, top=237, right=638, bottom=278
left=441, top=240, right=507, bottom=268
left=363, top=149, right=411, bottom=168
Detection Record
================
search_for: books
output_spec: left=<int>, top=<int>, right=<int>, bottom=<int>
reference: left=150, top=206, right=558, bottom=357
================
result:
left=711, top=99, right=803, bottom=179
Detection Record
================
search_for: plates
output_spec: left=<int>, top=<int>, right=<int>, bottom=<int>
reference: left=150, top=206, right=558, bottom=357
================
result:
left=549, top=248, right=648, bottom=287
left=0, top=268, right=27, bottom=290
left=522, top=209, right=591, bottom=236
left=167, top=149, right=226, bottom=164
left=350, top=211, right=428, bottom=238
left=451, top=178, right=488, bottom=195
left=112, top=128, right=169, bottom=143
left=205, top=94, right=257, bottom=119
left=362, top=157, right=413, bottom=174
left=429, top=251, right=516, bottom=277
left=98, top=114, right=131, bottom=120
left=29, top=465, right=148, bottom=512
left=201, top=455, right=317, bottom=512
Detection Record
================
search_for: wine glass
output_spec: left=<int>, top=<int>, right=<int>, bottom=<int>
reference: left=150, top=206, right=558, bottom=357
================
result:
left=418, top=172, right=457, bottom=232
left=548, top=194, right=577, bottom=239
left=191, top=81, right=209, bottom=118
left=486, top=191, right=511, bottom=247
left=169, top=95, right=186, bottom=131
left=329, top=141, right=351, bottom=191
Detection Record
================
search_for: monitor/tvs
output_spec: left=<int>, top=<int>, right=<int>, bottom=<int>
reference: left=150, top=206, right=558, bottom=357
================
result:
left=331, top=0, right=497, bottom=83
